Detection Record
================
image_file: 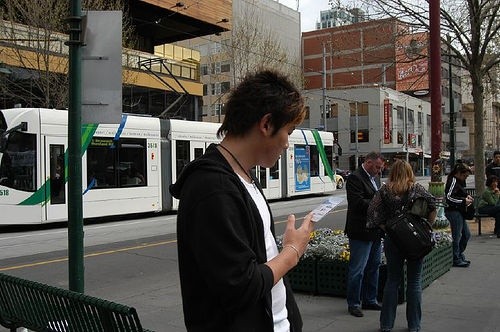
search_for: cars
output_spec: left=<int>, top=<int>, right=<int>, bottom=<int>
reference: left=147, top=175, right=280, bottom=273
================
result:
left=337, top=166, right=349, bottom=189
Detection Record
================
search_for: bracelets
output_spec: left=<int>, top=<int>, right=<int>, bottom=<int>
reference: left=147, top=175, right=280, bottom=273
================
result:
left=285, top=245, right=300, bottom=260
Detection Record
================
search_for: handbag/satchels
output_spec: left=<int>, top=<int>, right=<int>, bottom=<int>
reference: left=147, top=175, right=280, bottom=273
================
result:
left=386, top=213, right=436, bottom=261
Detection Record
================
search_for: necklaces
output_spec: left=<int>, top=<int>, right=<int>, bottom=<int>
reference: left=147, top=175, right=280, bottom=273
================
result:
left=219, top=144, right=255, bottom=184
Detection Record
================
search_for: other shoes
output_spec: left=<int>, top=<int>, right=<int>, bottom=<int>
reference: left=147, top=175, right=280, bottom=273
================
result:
left=348, top=306, right=363, bottom=317
left=464, top=260, right=471, bottom=264
left=453, top=262, right=470, bottom=267
left=361, top=303, right=381, bottom=310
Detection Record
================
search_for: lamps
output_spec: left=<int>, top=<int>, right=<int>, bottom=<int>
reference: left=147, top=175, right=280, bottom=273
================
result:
left=216, top=18, right=230, bottom=24
left=168, top=2, right=185, bottom=9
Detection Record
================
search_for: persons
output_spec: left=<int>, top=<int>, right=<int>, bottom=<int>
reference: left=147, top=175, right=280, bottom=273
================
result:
left=477, top=175, right=500, bottom=238
left=485, top=151, right=500, bottom=191
left=344, top=151, right=386, bottom=317
left=365, top=159, right=436, bottom=332
left=169, top=69, right=314, bottom=332
left=456, top=159, right=466, bottom=187
left=442, top=163, right=474, bottom=267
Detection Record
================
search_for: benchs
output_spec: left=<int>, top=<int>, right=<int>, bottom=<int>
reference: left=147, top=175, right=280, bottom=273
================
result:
left=0, top=273, right=155, bottom=332
left=473, top=194, right=492, bottom=236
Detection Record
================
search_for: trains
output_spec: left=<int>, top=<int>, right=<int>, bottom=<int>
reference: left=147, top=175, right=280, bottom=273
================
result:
left=0, top=106, right=337, bottom=230
